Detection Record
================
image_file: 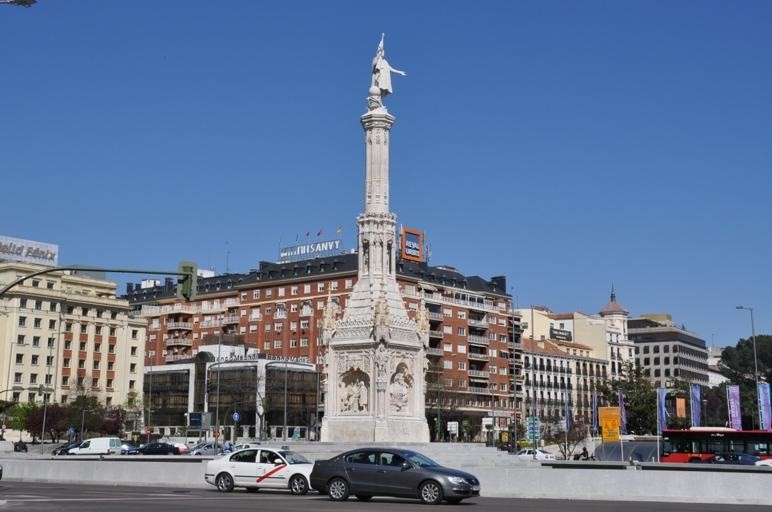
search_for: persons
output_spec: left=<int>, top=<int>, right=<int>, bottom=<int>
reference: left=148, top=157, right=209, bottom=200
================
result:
left=578, top=447, right=589, bottom=460
left=371, top=33, right=407, bottom=100
left=337, top=369, right=429, bottom=416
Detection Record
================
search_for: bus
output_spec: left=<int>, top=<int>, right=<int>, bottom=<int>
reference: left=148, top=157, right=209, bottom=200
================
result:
left=658, top=428, right=772, bottom=466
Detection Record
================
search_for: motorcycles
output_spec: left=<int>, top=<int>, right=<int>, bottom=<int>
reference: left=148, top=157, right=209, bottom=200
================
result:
left=572, top=453, right=598, bottom=460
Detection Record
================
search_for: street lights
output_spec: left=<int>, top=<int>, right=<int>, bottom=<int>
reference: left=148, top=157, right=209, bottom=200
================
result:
left=278, top=306, right=290, bottom=441
left=2, top=339, right=31, bottom=429
left=148, top=352, right=162, bottom=435
left=435, top=352, right=457, bottom=441
left=41, top=329, right=73, bottom=455
left=81, top=409, right=94, bottom=441
left=212, top=331, right=220, bottom=446
left=484, top=290, right=519, bottom=459
left=734, top=304, right=762, bottom=429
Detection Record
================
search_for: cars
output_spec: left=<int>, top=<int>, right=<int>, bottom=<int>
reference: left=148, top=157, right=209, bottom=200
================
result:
left=499, top=443, right=519, bottom=453
left=13, top=442, right=27, bottom=452
left=688, top=453, right=770, bottom=466
left=0, top=464, right=3, bottom=482
left=48, top=434, right=261, bottom=455
left=205, top=448, right=316, bottom=495
left=500, top=428, right=557, bottom=461
left=310, top=448, right=481, bottom=503
